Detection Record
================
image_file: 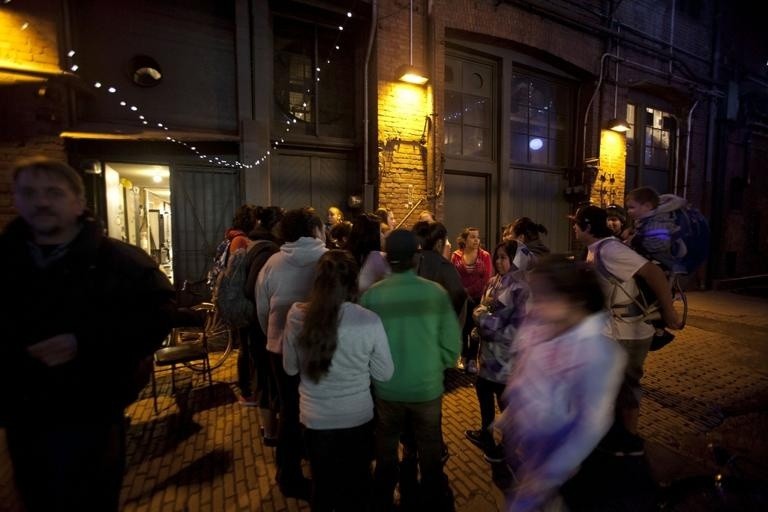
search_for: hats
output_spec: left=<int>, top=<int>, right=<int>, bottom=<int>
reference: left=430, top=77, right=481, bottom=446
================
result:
left=606, top=203, right=626, bottom=222
left=567, top=206, right=607, bottom=224
left=384, top=230, right=426, bottom=257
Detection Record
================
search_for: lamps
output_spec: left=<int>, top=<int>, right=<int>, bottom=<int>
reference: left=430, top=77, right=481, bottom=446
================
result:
left=607, top=25, right=632, bottom=137
left=397, top=0, right=431, bottom=85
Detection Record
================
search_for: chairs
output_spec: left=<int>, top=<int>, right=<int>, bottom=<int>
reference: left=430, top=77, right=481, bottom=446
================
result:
left=151, top=307, right=213, bottom=417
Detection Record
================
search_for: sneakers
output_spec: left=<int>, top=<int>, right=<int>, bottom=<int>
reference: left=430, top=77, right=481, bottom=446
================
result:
left=263, top=432, right=286, bottom=445
left=651, top=331, right=674, bottom=351
left=464, top=429, right=493, bottom=446
left=239, top=395, right=257, bottom=406
left=485, top=445, right=508, bottom=462
left=468, top=360, right=478, bottom=373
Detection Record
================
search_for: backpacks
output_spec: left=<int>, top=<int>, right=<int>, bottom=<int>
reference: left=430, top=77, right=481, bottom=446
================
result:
left=206, top=229, right=247, bottom=296
left=214, top=241, right=279, bottom=328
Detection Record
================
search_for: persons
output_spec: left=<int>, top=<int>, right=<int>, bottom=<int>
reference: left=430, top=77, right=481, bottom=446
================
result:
left=0, top=157, right=180, bottom=512
left=221, top=186, right=698, bottom=511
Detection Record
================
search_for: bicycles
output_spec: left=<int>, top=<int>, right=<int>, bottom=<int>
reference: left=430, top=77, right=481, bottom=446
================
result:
left=167, top=238, right=234, bottom=372
